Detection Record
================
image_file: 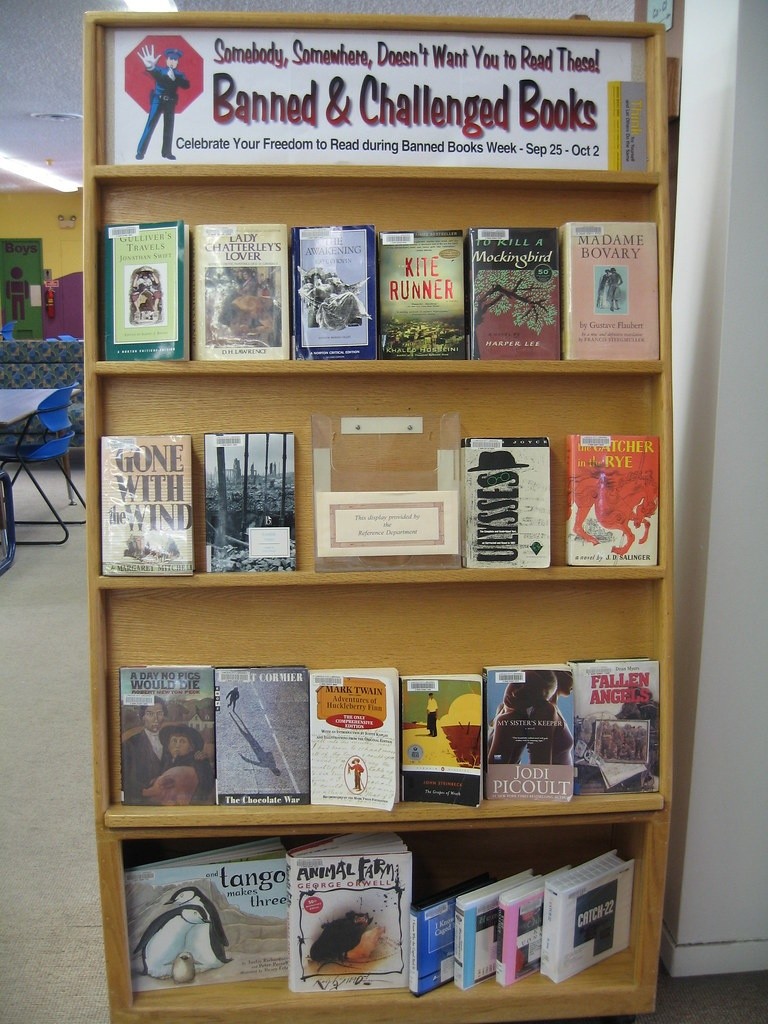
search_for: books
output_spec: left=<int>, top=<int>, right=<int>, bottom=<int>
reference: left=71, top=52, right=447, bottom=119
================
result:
left=124, top=834, right=633, bottom=999
left=100, top=226, right=658, bottom=361
left=118, top=664, right=661, bottom=805
left=100, top=435, right=658, bottom=574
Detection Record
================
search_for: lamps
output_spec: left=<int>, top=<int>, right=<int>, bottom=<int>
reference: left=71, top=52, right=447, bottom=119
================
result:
left=58, top=215, right=76, bottom=229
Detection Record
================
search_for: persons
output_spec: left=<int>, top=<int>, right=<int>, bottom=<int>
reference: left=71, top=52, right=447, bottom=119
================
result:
left=488, top=670, right=557, bottom=764
left=225, top=686, right=240, bottom=712
left=527, top=672, right=574, bottom=765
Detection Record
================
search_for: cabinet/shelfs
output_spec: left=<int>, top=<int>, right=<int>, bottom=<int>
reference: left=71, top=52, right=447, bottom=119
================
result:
left=83, top=9, right=675, bottom=1024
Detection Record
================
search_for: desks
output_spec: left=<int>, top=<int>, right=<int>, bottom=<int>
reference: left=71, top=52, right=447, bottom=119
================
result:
left=0, top=389, right=81, bottom=563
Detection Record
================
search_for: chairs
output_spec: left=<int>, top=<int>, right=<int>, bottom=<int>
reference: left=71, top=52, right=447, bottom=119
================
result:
left=46, top=335, right=79, bottom=342
left=1, top=382, right=86, bottom=575
left=0, top=320, right=18, bottom=340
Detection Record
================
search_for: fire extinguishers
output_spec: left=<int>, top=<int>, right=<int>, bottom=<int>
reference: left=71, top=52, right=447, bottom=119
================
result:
left=45, top=287, right=56, bottom=321
